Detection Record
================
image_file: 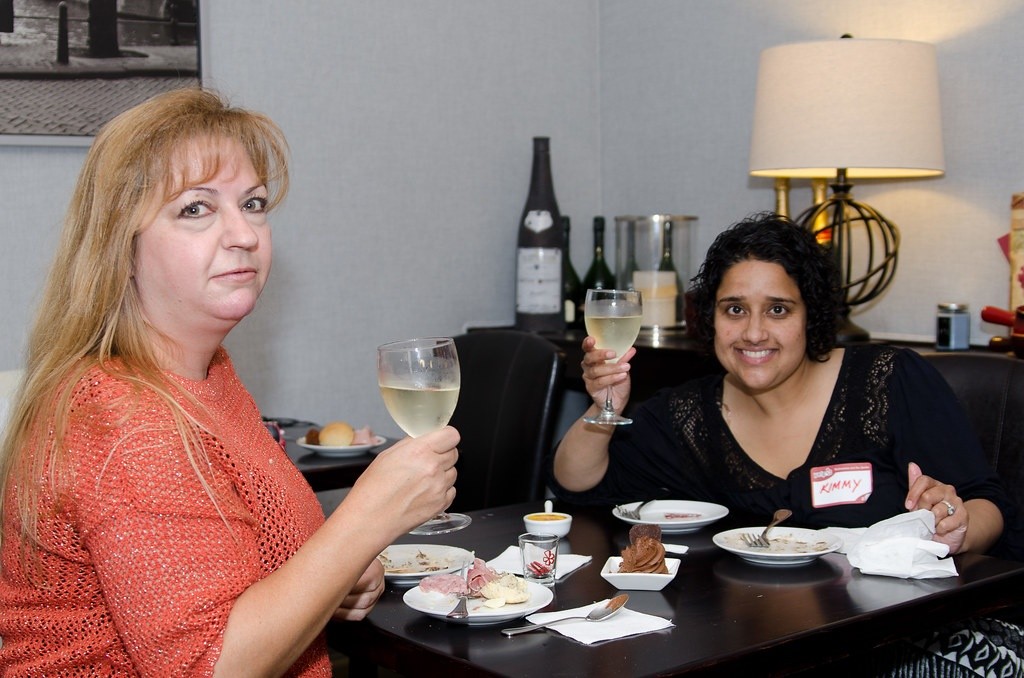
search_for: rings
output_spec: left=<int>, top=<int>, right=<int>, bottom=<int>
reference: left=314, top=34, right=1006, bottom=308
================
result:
left=938, top=498, right=954, bottom=514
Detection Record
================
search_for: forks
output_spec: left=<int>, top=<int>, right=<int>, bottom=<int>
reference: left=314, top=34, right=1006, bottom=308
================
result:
left=615, top=486, right=669, bottom=520
left=741, top=510, right=794, bottom=547
left=446, top=561, right=474, bottom=618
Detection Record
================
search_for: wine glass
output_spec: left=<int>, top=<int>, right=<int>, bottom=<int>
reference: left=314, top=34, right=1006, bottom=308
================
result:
left=378, top=336, right=474, bottom=537
left=583, top=288, right=642, bottom=426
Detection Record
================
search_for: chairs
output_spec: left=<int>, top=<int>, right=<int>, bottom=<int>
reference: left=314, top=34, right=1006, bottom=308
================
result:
left=924, top=354, right=1024, bottom=537
left=445, top=331, right=564, bottom=515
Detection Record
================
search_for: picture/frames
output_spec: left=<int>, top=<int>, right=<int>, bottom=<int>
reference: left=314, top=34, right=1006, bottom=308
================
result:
left=0, top=0, right=212, bottom=148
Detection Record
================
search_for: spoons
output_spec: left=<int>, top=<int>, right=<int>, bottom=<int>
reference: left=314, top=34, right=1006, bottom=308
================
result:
left=501, top=593, right=630, bottom=635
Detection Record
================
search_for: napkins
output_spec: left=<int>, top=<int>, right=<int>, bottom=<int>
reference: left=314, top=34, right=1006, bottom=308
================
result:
left=822, top=507, right=959, bottom=579
left=470, top=546, right=593, bottom=580
left=526, top=598, right=676, bottom=645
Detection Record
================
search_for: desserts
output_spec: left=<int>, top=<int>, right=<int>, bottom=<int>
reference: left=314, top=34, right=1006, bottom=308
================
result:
left=617, top=524, right=669, bottom=575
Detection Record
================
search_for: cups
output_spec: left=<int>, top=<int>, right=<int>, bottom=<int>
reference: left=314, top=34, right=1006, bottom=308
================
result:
left=519, top=533, right=559, bottom=586
left=523, top=511, right=572, bottom=538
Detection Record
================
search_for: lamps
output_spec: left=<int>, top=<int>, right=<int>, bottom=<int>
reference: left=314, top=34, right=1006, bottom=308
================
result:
left=750, top=34, right=944, bottom=348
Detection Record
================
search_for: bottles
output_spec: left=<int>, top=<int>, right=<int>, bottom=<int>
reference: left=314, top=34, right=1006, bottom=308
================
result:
left=515, top=137, right=686, bottom=331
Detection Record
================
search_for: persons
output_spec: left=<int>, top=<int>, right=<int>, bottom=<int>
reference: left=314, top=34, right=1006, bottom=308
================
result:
left=0, top=84, right=460, bottom=678
left=554, top=212, right=1024, bottom=678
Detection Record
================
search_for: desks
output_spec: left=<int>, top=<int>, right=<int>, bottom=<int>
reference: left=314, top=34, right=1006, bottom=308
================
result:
left=332, top=499, right=1024, bottom=678
left=263, top=417, right=401, bottom=493
left=470, top=325, right=1024, bottom=381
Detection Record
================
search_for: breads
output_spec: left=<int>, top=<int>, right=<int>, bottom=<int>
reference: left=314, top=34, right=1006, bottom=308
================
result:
left=318, top=421, right=355, bottom=446
left=480, top=575, right=529, bottom=608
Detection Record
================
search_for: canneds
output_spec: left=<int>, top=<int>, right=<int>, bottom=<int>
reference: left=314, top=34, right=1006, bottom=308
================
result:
left=935, top=302, right=971, bottom=350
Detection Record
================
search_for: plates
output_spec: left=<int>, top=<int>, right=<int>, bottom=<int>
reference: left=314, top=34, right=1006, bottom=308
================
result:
left=712, top=526, right=843, bottom=565
left=403, top=578, right=553, bottom=626
left=375, top=544, right=476, bottom=585
left=600, top=556, right=681, bottom=590
left=296, top=436, right=389, bottom=457
left=612, top=499, right=729, bottom=532
